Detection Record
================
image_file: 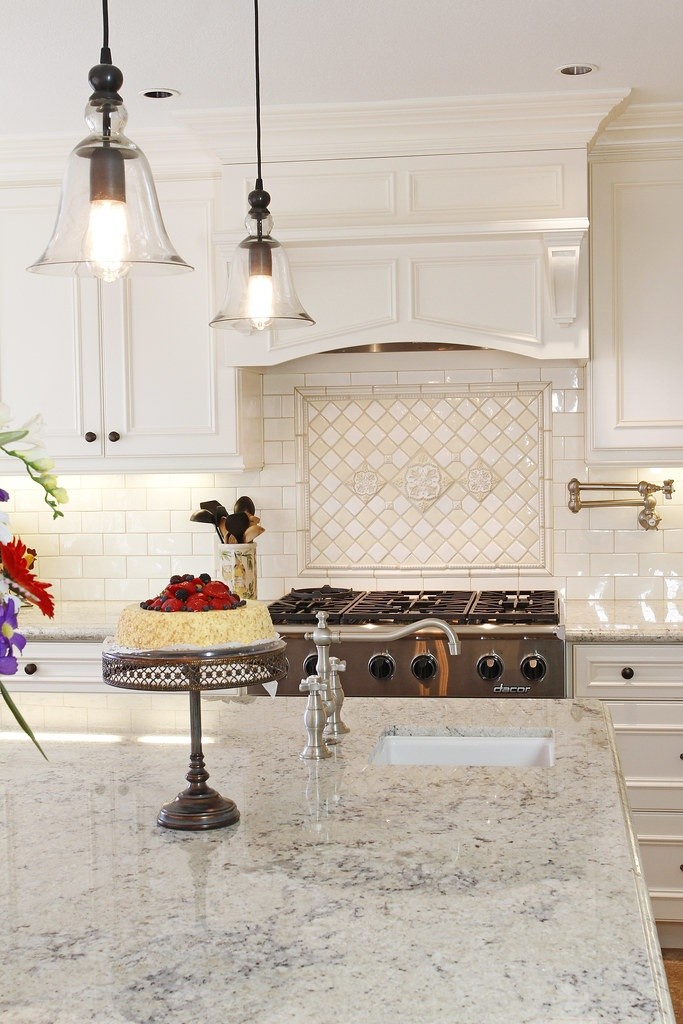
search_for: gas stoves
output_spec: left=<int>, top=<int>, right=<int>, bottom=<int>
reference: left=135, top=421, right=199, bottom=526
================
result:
left=245, top=584, right=567, bottom=699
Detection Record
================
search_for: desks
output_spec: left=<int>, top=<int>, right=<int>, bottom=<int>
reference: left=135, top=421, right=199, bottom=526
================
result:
left=99, top=642, right=289, bottom=832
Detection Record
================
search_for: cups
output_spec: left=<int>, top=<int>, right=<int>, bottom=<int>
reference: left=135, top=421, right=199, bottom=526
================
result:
left=216, top=543, right=257, bottom=600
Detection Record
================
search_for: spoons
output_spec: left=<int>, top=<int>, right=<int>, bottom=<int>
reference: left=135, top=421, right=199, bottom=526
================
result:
left=190, top=496, right=265, bottom=544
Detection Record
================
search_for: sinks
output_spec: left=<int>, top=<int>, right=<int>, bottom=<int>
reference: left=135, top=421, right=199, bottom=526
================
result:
left=365, top=726, right=554, bottom=768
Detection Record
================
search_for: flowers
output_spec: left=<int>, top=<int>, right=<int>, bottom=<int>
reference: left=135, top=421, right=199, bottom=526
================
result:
left=0, top=415, right=70, bottom=759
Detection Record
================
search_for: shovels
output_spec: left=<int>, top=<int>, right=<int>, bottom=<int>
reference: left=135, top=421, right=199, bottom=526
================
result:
left=214, top=506, right=230, bottom=527
left=200, top=500, right=227, bottom=518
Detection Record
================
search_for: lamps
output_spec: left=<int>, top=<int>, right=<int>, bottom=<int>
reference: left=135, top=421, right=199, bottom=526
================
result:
left=27, top=0, right=194, bottom=283
left=208, top=0, right=315, bottom=328
left=567, top=478, right=676, bottom=530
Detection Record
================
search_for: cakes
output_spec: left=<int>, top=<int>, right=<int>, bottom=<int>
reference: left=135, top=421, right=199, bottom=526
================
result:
left=115, top=573, right=275, bottom=651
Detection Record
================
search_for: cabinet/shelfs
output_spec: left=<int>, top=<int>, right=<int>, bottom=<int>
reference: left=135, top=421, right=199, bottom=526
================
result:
left=571, top=644, right=683, bottom=948
left=585, top=105, right=683, bottom=467
left=0, top=129, right=266, bottom=476
left=212, top=149, right=591, bottom=374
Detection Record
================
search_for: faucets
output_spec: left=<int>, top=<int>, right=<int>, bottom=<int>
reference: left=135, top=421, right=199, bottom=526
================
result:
left=304, top=610, right=462, bottom=734
